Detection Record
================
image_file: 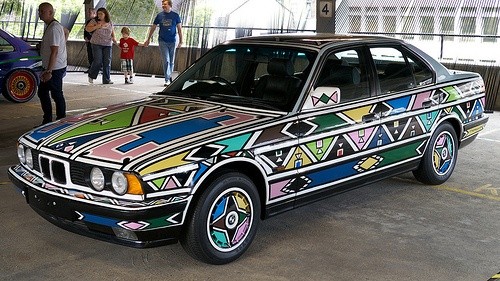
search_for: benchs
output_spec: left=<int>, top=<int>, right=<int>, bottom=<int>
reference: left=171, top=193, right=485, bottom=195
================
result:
left=324, top=60, right=414, bottom=85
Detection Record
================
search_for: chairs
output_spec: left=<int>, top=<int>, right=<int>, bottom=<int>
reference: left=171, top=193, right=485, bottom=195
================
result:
left=255, top=59, right=301, bottom=104
left=322, top=64, right=360, bottom=102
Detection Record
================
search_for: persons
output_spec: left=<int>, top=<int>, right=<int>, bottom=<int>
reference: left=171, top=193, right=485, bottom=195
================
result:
left=144, top=0, right=183, bottom=86
left=34, top=2, right=69, bottom=129
left=84, top=8, right=117, bottom=84
left=113, top=27, right=149, bottom=84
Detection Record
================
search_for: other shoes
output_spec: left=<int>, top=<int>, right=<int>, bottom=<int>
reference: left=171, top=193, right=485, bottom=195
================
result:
left=103, top=80, right=114, bottom=84
left=164, top=79, right=171, bottom=86
left=125, top=77, right=135, bottom=84
left=88, top=76, right=94, bottom=83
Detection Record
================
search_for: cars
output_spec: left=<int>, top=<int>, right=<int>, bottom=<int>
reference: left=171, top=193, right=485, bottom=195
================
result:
left=0, top=28, right=46, bottom=104
left=7, top=32, right=489, bottom=266
